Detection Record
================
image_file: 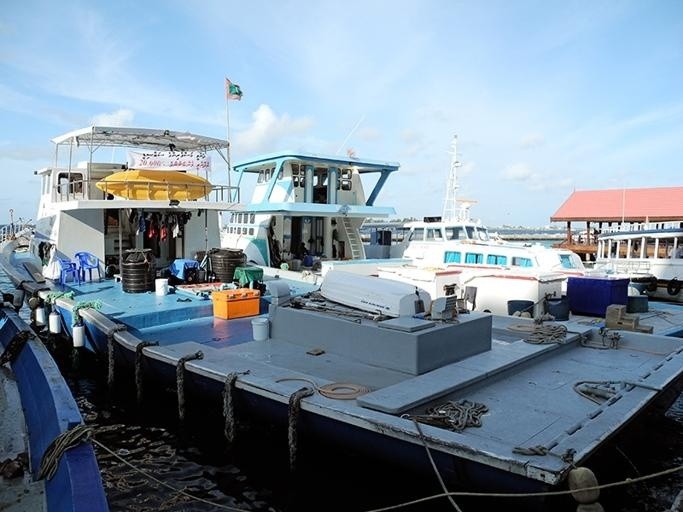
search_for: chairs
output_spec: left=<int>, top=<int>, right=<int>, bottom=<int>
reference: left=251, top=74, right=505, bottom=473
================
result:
left=57, top=257, right=81, bottom=287
left=75, top=251, right=101, bottom=284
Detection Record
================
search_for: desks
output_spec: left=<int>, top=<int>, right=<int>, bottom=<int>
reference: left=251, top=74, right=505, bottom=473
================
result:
left=233, top=266, right=263, bottom=289
left=169, top=259, right=200, bottom=284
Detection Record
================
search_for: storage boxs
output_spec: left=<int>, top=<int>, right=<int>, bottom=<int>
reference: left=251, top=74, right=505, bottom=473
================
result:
left=627, top=295, right=649, bottom=312
left=212, top=288, right=260, bottom=319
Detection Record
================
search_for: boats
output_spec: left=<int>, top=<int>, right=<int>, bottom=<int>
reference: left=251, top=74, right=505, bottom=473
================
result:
left=357, top=134, right=683, bottom=297
left=95, top=169, right=213, bottom=200
left=0, top=126, right=683, bottom=512
left=0, top=292, right=110, bottom=512
left=219, top=150, right=683, bottom=337
left=319, top=267, right=432, bottom=318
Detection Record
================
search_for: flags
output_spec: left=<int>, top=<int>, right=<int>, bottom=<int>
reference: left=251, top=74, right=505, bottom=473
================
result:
left=225, top=78, right=241, bottom=101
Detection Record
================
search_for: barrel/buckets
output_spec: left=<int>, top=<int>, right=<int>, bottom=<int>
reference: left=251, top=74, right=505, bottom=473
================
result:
left=155, top=278, right=168, bottom=297
left=508, top=300, right=534, bottom=317
left=210, top=249, right=247, bottom=283
left=251, top=318, right=269, bottom=341
left=543, top=296, right=570, bottom=321
left=122, top=251, right=154, bottom=293
left=305, top=258, right=313, bottom=267
